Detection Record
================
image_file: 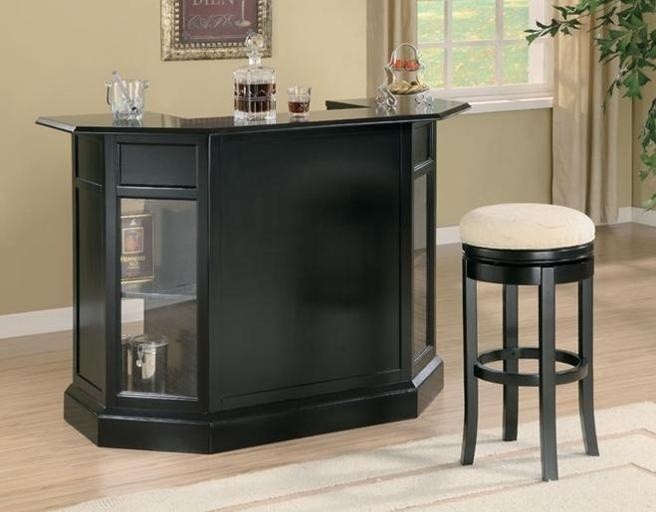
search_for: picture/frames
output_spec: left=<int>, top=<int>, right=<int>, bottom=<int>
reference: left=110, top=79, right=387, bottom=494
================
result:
left=159, top=0, right=273, bottom=61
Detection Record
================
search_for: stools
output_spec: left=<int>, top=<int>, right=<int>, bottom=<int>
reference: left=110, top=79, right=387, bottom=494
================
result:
left=460, top=203, right=600, bottom=481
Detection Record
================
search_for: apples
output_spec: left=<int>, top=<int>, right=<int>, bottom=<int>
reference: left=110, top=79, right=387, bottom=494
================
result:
left=393, top=59, right=418, bottom=71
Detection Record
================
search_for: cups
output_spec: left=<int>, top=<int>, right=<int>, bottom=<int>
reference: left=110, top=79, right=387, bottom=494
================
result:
left=288, top=86, right=311, bottom=118
left=107, top=80, right=150, bottom=121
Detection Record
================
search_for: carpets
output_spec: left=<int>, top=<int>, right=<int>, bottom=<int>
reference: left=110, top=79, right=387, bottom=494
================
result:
left=49, top=401, right=656, bottom=512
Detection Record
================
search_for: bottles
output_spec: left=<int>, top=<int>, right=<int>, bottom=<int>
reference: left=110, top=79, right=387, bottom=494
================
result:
left=234, top=32, right=277, bottom=123
